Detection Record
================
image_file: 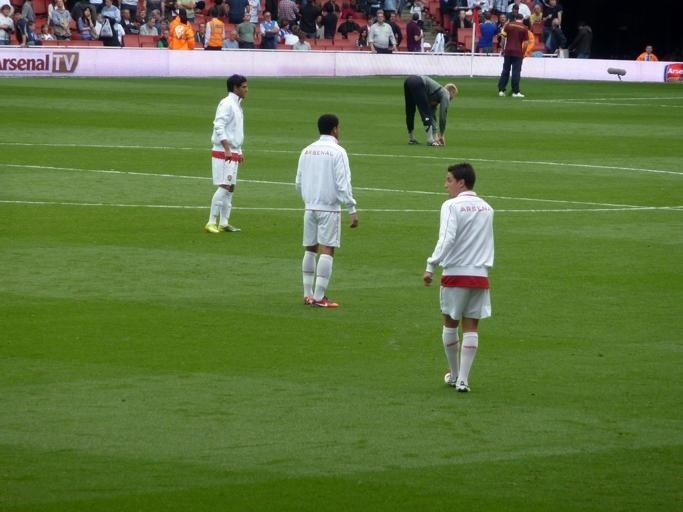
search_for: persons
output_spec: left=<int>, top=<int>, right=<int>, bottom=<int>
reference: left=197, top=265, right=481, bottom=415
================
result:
left=498, top=14, right=529, bottom=97
left=569, top=25, right=593, bottom=58
left=295, top=115, right=358, bottom=307
left=422, top=163, right=495, bottom=392
left=404, top=75, right=458, bottom=147
left=205, top=74, right=248, bottom=233
left=0, top=0, right=569, bottom=58
left=636, top=45, right=658, bottom=61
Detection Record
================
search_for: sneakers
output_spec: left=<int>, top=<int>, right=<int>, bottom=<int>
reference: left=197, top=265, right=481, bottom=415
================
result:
left=205, top=222, right=220, bottom=233
left=305, top=296, right=339, bottom=307
left=409, top=139, right=441, bottom=146
left=445, top=373, right=457, bottom=386
left=218, top=224, right=241, bottom=232
left=455, top=380, right=470, bottom=391
left=499, top=91, right=524, bottom=97
